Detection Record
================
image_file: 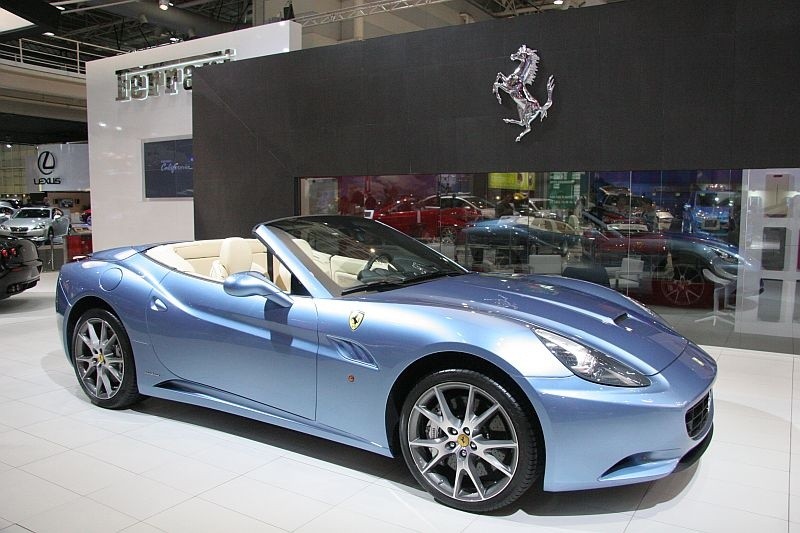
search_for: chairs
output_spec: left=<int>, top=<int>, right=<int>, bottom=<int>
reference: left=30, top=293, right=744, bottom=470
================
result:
left=209, top=236, right=267, bottom=281
left=277, top=239, right=330, bottom=291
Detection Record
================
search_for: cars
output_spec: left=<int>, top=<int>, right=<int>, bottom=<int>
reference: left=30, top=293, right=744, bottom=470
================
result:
left=0, top=197, right=20, bottom=224
left=0, top=228, right=44, bottom=301
left=79, top=208, right=91, bottom=225
left=0, top=206, right=71, bottom=247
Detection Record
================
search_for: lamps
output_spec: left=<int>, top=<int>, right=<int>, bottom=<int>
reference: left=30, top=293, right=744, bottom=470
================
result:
left=187, top=27, right=197, bottom=40
left=139, top=13, right=148, bottom=27
left=158, top=0, right=170, bottom=10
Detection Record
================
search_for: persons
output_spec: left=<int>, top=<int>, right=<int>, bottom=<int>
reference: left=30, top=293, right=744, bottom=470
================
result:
left=697, top=194, right=725, bottom=235
left=565, top=182, right=687, bottom=233
left=495, top=192, right=513, bottom=218
left=338, top=183, right=465, bottom=214
left=24, top=197, right=50, bottom=208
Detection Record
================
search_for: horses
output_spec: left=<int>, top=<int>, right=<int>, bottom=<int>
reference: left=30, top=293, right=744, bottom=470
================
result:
left=492, top=44, right=555, bottom=142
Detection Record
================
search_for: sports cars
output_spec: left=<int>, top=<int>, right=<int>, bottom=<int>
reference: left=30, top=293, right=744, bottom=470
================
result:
left=369, top=173, right=765, bottom=308
left=55, top=212, right=717, bottom=513
